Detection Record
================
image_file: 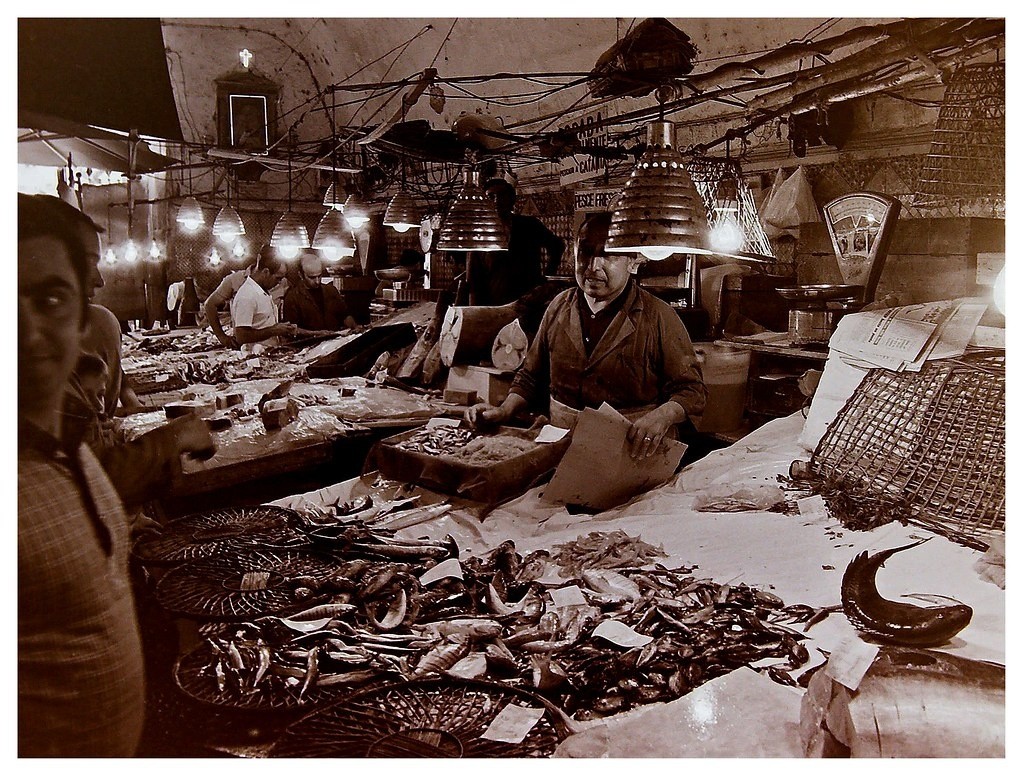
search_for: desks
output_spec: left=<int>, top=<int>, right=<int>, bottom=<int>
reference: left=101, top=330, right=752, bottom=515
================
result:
left=142, top=469, right=487, bottom=758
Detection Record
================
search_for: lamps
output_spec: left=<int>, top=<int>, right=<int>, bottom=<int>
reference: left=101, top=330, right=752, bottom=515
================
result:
left=311, top=90, right=357, bottom=261
left=685, top=140, right=778, bottom=264
left=436, top=168, right=510, bottom=252
left=342, top=194, right=371, bottom=228
left=269, top=130, right=310, bottom=258
left=604, top=93, right=711, bottom=260
left=323, top=181, right=347, bottom=211
left=175, top=152, right=206, bottom=230
left=382, top=155, right=422, bottom=233
left=212, top=163, right=246, bottom=242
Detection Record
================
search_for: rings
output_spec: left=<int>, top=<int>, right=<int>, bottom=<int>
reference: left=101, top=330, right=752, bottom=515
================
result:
left=644, top=437, right=651, bottom=440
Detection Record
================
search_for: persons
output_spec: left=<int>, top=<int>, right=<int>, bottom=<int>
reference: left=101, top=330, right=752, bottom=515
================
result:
left=205, top=245, right=298, bottom=355
left=841, top=235, right=848, bottom=254
left=18, top=190, right=145, bottom=758
left=33, top=194, right=217, bottom=541
left=854, top=236, right=866, bottom=251
left=283, top=255, right=365, bottom=352
left=464, top=213, right=705, bottom=515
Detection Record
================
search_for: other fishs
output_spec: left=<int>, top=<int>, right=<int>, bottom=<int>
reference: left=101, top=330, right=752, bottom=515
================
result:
left=195, top=488, right=976, bottom=759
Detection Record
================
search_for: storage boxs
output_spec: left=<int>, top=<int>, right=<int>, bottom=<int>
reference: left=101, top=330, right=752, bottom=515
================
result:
left=306, top=322, right=418, bottom=379
left=360, top=415, right=572, bottom=504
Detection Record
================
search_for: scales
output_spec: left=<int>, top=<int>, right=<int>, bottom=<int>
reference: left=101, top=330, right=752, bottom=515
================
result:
left=374, top=214, right=446, bottom=302
left=774, top=190, right=902, bottom=344
